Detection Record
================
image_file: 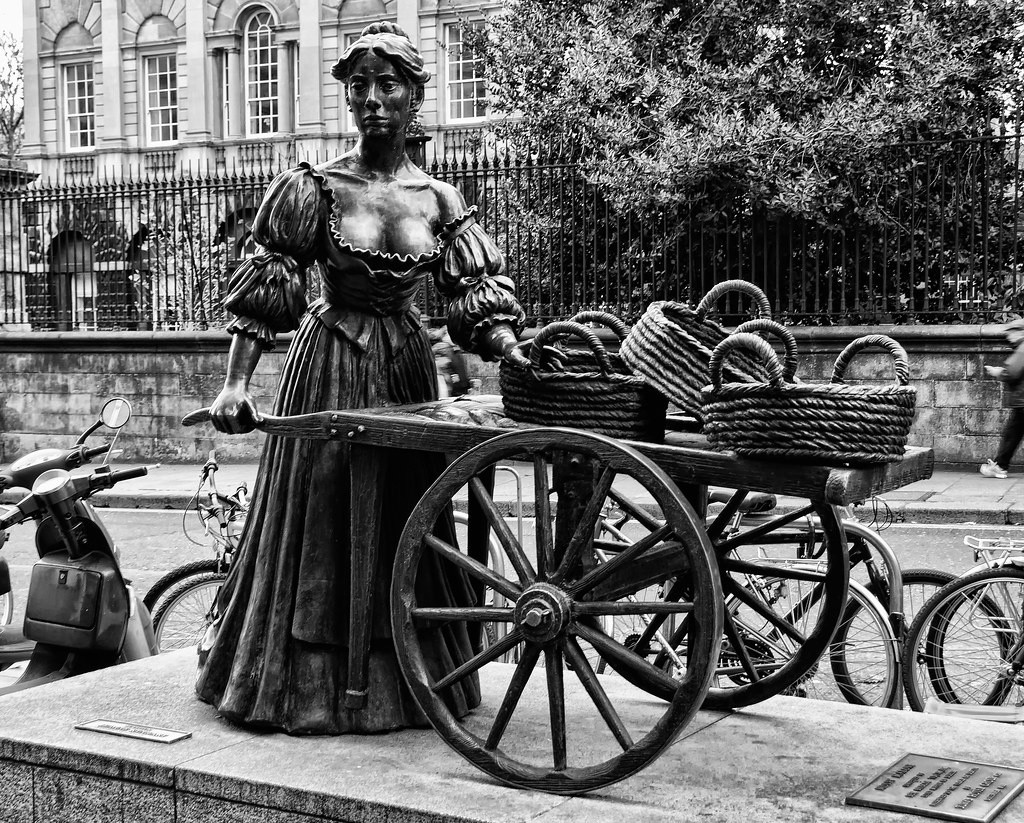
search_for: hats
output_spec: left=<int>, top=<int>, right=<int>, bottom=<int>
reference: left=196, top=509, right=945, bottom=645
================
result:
left=1004, top=319, right=1024, bottom=330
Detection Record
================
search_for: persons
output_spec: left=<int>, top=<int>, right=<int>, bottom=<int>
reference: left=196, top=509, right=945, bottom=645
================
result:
left=193, top=19, right=567, bottom=738
left=980, top=318, right=1024, bottom=477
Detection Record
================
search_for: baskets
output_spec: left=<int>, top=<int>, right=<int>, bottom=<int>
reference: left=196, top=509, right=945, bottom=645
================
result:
left=617, top=280, right=798, bottom=422
left=498, top=311, right=670, bottom=443
left=700, top=331, right=918, bottom=462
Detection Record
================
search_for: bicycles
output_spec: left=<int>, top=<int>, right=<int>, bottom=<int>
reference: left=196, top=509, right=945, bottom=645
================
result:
left=143, top=448, right=497, bottom=662
left=513, top=488, right=1023, bottom=718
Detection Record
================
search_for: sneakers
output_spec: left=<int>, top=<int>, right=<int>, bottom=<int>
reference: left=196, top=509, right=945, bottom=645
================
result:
left=980, top=458, right=1007, bottom=479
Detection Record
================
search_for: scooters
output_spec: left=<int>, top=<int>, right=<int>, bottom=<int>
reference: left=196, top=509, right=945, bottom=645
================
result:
left=0, top=428, right=158, bottom=695
left=0, top=397, right=132, bottom=670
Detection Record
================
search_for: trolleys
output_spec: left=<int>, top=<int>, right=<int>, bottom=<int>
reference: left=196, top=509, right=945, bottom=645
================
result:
left=180, top=396, right=938, bottom=797
